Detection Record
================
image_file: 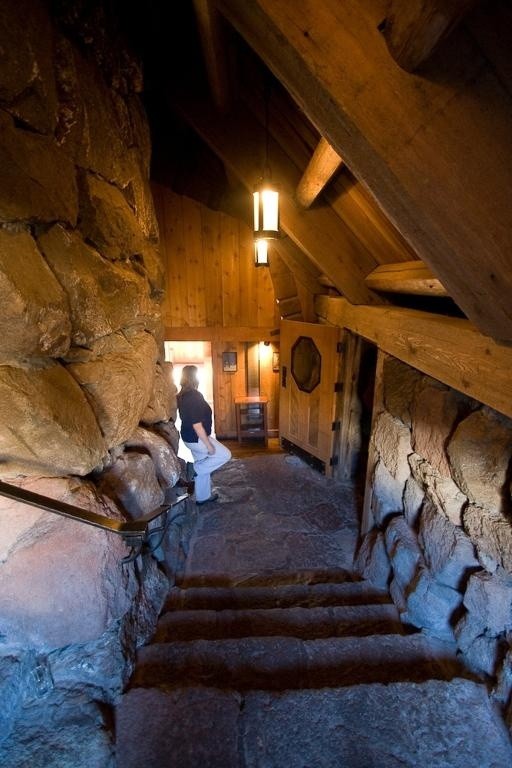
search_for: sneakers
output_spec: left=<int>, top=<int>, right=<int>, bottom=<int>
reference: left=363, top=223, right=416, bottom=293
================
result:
left=196, top=493, right=218, bottom=505
left=187, top=462, right=197, bottom=482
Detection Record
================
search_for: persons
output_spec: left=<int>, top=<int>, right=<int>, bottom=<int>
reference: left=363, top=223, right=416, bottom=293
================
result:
left=177, top=365, right=232, bottom=504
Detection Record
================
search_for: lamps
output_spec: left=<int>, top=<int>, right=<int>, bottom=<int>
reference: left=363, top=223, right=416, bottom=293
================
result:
left=253, top=85, right=281, bottom=267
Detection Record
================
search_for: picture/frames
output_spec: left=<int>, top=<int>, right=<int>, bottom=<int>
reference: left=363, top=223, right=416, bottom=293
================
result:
left=273, top=352, right=280, bottom=371
left=222, top=352, right=237, bottom=372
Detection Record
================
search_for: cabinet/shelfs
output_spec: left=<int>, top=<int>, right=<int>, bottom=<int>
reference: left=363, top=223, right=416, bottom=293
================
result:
left=235, top=396, right=268, bottom=445
